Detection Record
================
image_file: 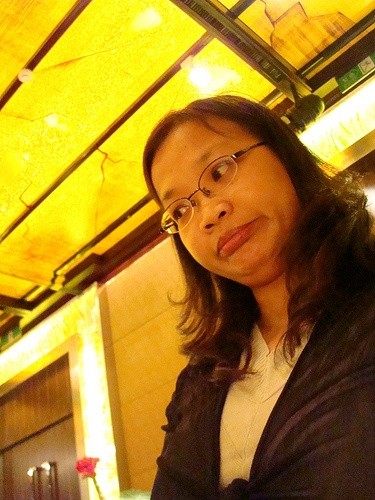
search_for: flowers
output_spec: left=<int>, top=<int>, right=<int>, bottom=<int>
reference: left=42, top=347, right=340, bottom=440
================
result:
left=75, top=456, right=106, bottom=500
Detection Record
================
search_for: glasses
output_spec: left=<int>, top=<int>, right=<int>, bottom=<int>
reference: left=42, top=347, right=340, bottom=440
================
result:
left=129, top=141, right=267, bottom=236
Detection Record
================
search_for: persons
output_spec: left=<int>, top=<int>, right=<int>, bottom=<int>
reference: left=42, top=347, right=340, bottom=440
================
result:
left=142, top=94, right=375, bottom=500
